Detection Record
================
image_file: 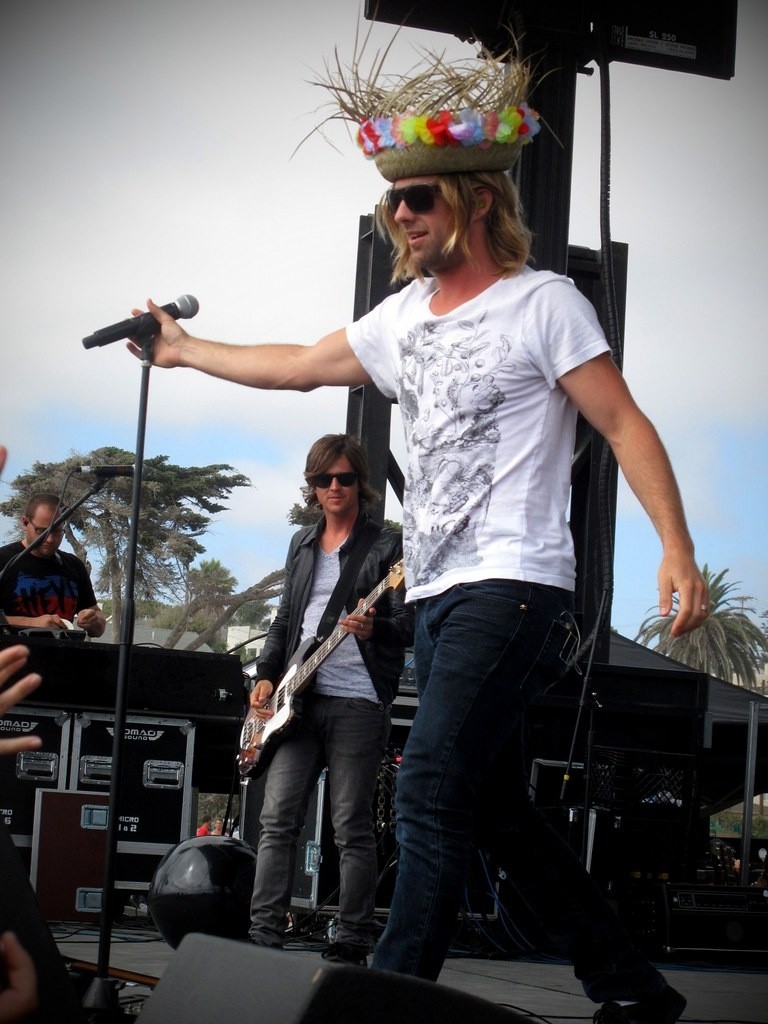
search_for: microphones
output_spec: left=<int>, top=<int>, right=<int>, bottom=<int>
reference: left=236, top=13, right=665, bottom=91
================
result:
left=76, top=463, right=149, bottom=479
left=82, top=295, right=199, bottom=349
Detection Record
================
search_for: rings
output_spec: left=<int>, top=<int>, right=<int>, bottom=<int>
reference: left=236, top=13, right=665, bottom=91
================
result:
left=360, top=622, right=364, bottom=630
left=701, top=604, right=707, bottom=611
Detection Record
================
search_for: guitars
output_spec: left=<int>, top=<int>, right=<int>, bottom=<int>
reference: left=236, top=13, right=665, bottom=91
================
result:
left=236, top=559, right=405, bottom=780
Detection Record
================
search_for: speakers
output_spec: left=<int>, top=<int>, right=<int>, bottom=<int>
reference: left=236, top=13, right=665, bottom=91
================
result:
left=0, top=812, right=81, bottom=1024
left=134, top=931, right=539, bottom=1024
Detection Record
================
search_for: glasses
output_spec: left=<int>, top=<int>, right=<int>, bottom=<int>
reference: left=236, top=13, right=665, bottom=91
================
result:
left=311, top=471, right=357, bottom=489
left=29, top=520, right=66, bottom=536
left=386, top=184, right=442, bottom=214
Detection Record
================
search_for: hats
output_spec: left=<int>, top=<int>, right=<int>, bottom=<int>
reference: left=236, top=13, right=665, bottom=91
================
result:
left=290, top=2, right=565, bottom=182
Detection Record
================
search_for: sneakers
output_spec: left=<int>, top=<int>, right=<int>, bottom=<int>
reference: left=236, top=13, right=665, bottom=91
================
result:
left=321, top=941, right=367, bottom=966
left=592, top=985, right=687, bottom=1024
left=248, top=939, right=284, bottom=951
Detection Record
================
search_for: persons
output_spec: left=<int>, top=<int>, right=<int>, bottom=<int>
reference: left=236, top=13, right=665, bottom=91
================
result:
left=0, top=645, right=43, bottom=1024
left=197, top=815, right=212, bottom=836
left=0, top=493, right=106, bottom=638
left=247, top=434, right=416, bottom=969
left=210, top=818, right=229, bottom=836
left=126, top=169, right=710, bottom=1024
